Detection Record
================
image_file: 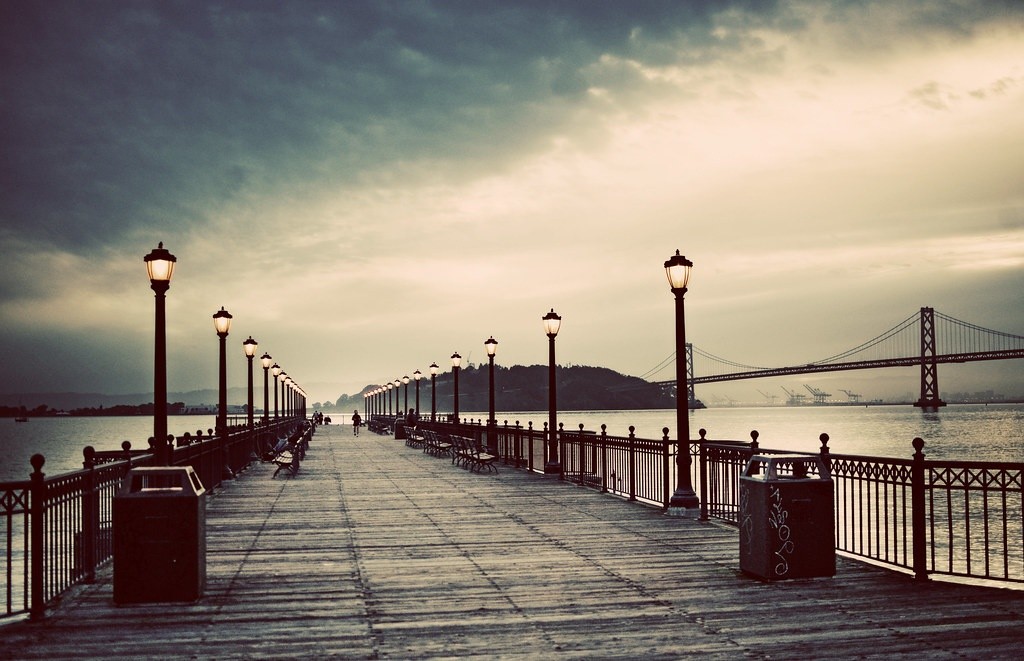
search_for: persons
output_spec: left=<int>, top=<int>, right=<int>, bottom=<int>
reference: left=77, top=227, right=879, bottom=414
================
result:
left=323, top=415, right=331, bottom=425
left=264, top=420, right=310, bottom=456
left=319, top=412, right=323, bottom=424
left=352, top=410, right=362, bottom=436
left=406, top=408, right=418, bottom=431
left=313, top=410, right=318, bottom=423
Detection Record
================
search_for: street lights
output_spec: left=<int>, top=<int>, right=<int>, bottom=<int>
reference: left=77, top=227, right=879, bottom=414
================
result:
left=363, top=393, right=368, bottom=422
left=289, top=380, right=295, bottom=417
left=279, top=369, right=286, bottom=420
left=484, top=335, right=500, bottom=461
left=394, top=378, right=401, bottom=418
left=387, top=382, right=393, bottom=418
left=377, top=387, right=382, bottom=418
left=542, top=307, right=562, bottom=474
left=450, top=352, right=462, bottom=436
left=402, top=374, right=410, bottom=423
left=243, top=335, right=258, bottom=461
left=143, top=242, right=179, bottom=488
left=260, top=352, right=273, bottom=451
left=212, top=306, right=235, bottom=480
left=413, top=369, right=422, bottom=422
left=381, top=384, right=387, bottom=418
left=370, top=391, right=374, bottom=420
left=374, top=388, right=378, bottom=417
left=662, top=248, right=700, bottom=510
left=293, top=383, right=307, bottom=418
left=367, top=391, right=371, bottom=421
left=270, top=362, right=279, bottom=445
left=285, top=376, right=291, bottom=418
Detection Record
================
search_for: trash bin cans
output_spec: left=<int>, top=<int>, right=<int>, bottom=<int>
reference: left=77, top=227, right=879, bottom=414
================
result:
left=394, top=418, right=406, bottom=439
left=112, top=466, right=208, bottom=602
left=303, top=421, right=312, bottom=441
left=739, top=454, right=836, bottom=580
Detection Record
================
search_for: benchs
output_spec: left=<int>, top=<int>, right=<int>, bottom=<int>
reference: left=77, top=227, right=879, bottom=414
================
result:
left=367, top=419, right=389, bottom=435
left=421, top=429, right=454, bottom=458
left=450, top=435, right=499, bottom=474
left=403, top=426, right=425, bottom=448
left=272, top=420, right=316, bottom=479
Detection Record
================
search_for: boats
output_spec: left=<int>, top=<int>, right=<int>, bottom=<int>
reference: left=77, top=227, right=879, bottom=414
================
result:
left=14, top=415, right=30, bottom=423
